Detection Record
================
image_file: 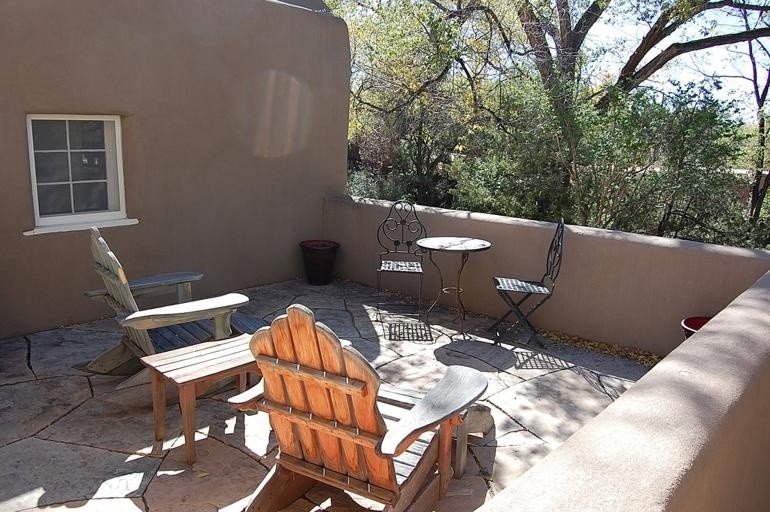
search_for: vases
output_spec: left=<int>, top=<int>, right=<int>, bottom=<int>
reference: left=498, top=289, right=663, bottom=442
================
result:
left=680, top=315, right=717, bottom=343
left=299, top=240, right=341, bottom=286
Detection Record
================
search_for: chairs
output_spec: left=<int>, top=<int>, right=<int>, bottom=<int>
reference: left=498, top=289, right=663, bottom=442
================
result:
left=486, top=218, right=564, bottom=351
left=79, top=227, right=273, bottom=410
left=376, top=201, right=427, bottom=321
left=226, top=302, right=494, bottom=512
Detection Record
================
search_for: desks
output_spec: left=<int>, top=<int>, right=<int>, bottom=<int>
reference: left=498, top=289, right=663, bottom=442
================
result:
left=414, top=236, right=491, bottom=342
